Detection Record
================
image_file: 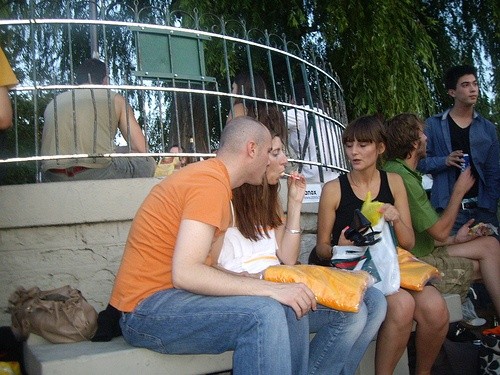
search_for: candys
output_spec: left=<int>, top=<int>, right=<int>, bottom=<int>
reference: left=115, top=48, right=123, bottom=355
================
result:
left=281, top=172, right=299, bottom=179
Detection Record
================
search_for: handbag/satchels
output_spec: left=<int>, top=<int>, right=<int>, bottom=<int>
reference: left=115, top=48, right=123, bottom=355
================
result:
left=329, top=209, right=400, bottom=296
left=407, top=322, right=481, bottom=375
left=3, top=285, right=99, bottom=344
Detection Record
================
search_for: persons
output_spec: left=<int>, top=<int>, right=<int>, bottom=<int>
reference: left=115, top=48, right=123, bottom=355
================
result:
left=0, top=47, right=19, bottom=130
left=39, top=58, right=157, bottom=182
left=316, top=117, right=451, bottom=375
left=375, top=64, right=500, bottom=327
left=218, top=114, right=387, bottom=375
left=168, top=69, right=347, bottom=182
left=108, top=117, right=317, bottom=375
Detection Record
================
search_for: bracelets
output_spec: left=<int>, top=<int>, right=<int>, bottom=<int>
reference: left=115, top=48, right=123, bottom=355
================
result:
left=258, top=272, right=263, bottom=281
left=330, top=248, right=336, bottom=257
left=284, top=226, right=304, bottom=234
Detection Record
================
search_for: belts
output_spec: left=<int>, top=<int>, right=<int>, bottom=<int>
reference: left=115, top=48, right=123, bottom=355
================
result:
left=50, top=166, right=87, bottom=174
left=460, top=202, right=478, bottom=211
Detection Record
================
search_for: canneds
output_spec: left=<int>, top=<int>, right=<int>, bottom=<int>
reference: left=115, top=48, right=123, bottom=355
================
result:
left=459, top=154, right=469, bottom=172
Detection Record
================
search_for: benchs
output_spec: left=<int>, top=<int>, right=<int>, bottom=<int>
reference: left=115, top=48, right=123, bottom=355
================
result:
left=20, top=290, right=464, bottom=375
